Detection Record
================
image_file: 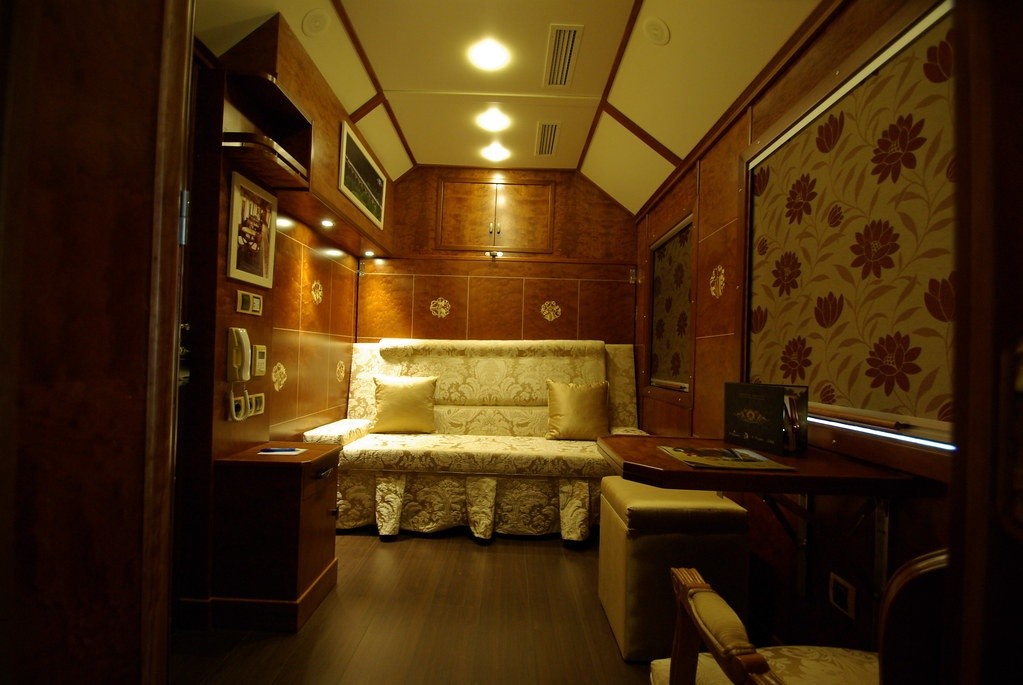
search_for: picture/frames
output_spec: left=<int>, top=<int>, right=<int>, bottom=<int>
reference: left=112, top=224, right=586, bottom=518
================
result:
left=338, top=120, right=387, bottom=230
left=227, top=171, right=277, bottom=290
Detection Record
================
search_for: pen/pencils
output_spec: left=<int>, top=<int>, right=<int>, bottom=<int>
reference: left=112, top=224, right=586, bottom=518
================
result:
left=727, top=448, right=743, bottom=462
left=259, top=448, right=295, bottom=452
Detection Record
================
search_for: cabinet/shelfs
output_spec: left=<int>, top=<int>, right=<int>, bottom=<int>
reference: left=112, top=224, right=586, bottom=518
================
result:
left=436, top=176, right=555, bottom=253
left=212, top=442, right=342, bottom=633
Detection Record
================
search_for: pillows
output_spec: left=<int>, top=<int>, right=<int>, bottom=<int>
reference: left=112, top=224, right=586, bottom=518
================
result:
left=545, top=379, right=611, bottom=440
left=368, top=374, right=439, bottom=433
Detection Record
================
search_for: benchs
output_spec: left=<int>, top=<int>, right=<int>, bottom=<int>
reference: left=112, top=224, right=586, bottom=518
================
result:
left=303, top=338, right=646, bottom=542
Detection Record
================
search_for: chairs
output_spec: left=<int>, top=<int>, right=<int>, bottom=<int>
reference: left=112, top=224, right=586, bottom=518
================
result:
left=648, top=547, right=947, bottom=685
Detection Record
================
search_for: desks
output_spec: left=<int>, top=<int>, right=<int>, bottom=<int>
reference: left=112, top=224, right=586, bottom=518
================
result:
left=597, top=435, right=907, bottom=643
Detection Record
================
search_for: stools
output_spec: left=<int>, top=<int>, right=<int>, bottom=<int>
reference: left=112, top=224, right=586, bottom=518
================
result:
left=598, top=476, right=749, bottom=661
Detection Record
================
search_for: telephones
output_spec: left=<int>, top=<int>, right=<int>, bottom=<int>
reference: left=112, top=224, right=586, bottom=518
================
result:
left=226, top=326, right=251, bottom=383
